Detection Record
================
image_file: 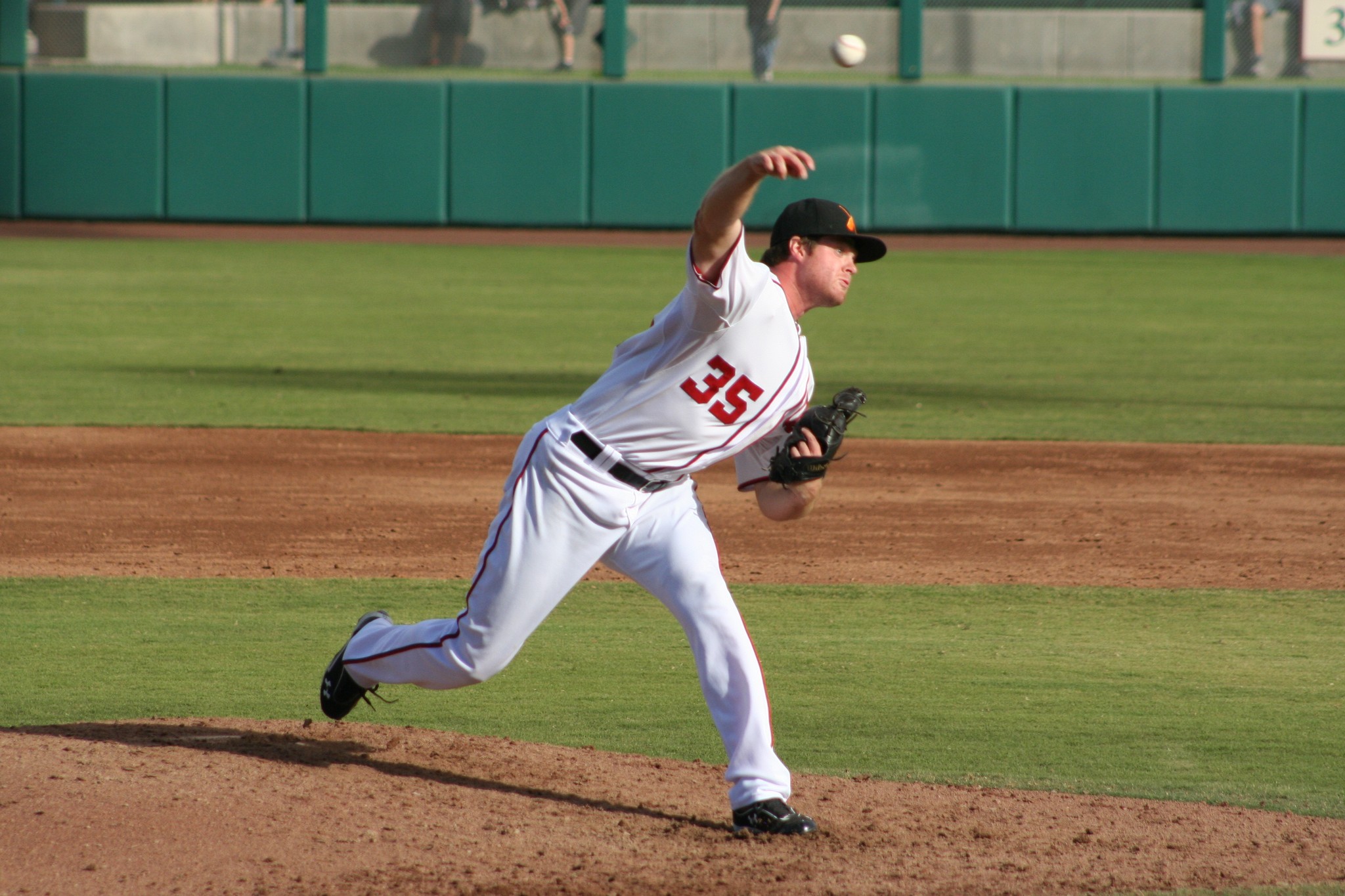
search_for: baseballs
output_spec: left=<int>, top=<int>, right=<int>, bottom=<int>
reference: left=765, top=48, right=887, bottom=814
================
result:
left=831, top=34, right=867, bottom=67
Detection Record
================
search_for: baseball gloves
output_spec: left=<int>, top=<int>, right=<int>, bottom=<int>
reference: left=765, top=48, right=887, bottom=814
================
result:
left=769, top=387, right=868, bottom=483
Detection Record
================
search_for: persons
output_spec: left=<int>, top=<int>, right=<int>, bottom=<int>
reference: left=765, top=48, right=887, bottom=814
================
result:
left=425, top=0, right=476, bottom=73
left=319, top=145, right=886, bottom=838
left=747, top=0, right=788, bottom=86
left=541, top=0, right=593, bottom=74
left=1227, top=0, right=1318, bottom=79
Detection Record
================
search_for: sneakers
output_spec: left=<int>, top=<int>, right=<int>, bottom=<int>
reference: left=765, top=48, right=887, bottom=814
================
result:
left=321, top=611, right=399, bottom=719
left=733, top=799, right=818, bottom=835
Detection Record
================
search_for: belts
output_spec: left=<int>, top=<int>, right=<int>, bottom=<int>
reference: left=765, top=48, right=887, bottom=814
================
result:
left=573, top=431, right=672, bottom=493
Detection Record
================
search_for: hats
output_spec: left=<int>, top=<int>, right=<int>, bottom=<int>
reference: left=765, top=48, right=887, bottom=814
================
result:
left=770, top=198, right=888, bottom=264
